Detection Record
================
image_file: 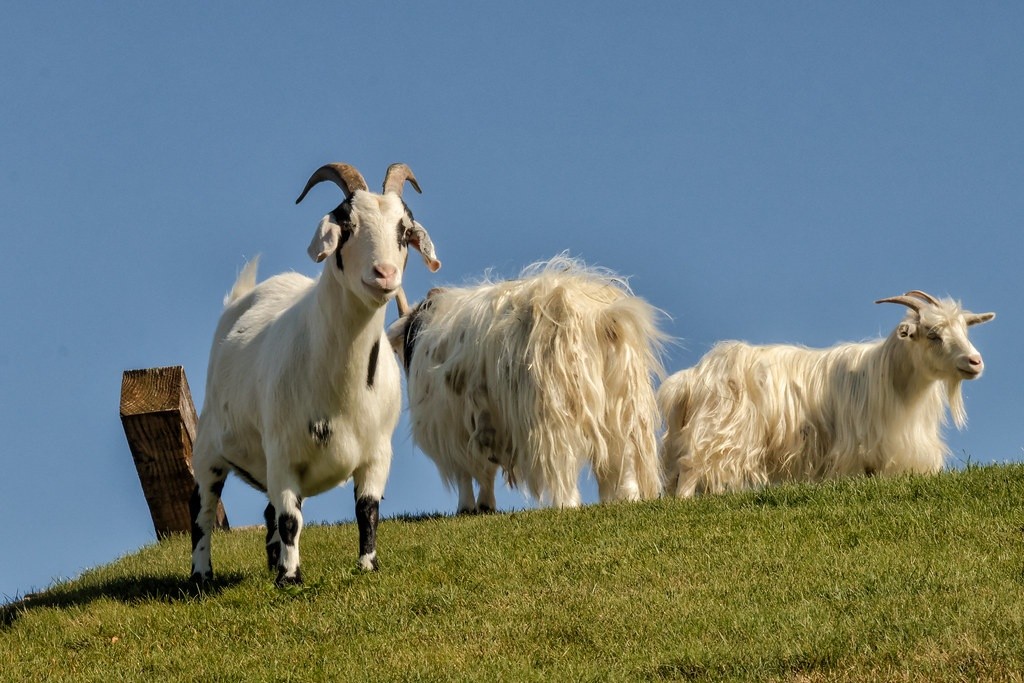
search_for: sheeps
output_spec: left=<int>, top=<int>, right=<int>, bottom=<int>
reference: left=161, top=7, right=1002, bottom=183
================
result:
left=654, top=290, right=996, bottom=498
left=176, top=161, right=442, bottom=590
left=381, top=248, right=687, bottom=512
